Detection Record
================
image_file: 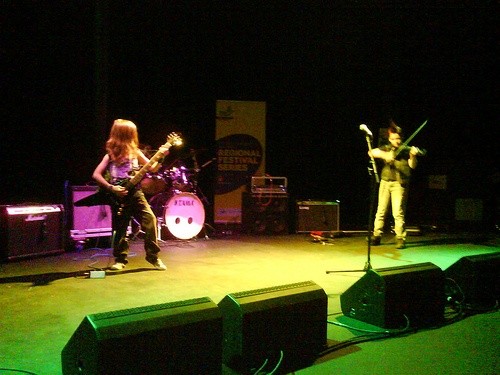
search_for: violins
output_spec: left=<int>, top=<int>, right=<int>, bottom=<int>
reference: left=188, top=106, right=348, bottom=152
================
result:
left=380, top=144, right=427, bottom=156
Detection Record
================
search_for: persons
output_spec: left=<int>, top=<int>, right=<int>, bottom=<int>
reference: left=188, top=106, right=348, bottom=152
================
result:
left=368, top=126, right=418, bottom=249
left=92, top=119, right=169, bottom=269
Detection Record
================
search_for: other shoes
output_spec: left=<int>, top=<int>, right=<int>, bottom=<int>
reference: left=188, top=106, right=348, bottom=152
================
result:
left=395, top=239, right=406, bottom=249
left=366, top=235, right=381, bottom=245
left=145, top=255, right=167, bottom=270
left=110, top=262, right=126, bottom=270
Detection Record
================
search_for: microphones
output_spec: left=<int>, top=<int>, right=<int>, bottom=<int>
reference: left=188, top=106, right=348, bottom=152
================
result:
left=102, top=205, right=107, bottom=217
left=322, top=208, right=326, bottom=223
left=359, top=124, right=373, bottom=136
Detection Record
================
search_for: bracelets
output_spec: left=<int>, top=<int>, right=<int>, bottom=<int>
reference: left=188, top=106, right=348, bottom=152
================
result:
left=110, top=186, right=112, bottom=189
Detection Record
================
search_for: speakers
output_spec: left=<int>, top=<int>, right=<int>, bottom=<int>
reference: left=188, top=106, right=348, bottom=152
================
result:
left=0, top=203, right=65, bottom=263
left=340, top=251, right=500, bottom=329
left=61, top=281, right=328, bottom=375
left=295, top=200, right=339, bottom=234
left=241, top=192, right=291, bottom=236
left=64, top=185, right=113, bottom=230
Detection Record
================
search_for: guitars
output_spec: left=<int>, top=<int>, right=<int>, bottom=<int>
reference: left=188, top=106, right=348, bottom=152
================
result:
left=72, top=132, right=184, bottom=221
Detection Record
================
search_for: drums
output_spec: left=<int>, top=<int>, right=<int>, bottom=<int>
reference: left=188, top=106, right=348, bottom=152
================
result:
left=148, top=192, right=206, bottom=240
